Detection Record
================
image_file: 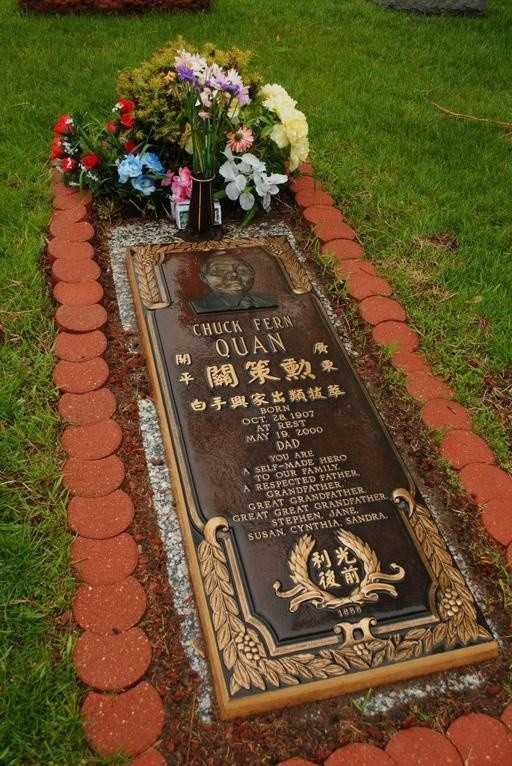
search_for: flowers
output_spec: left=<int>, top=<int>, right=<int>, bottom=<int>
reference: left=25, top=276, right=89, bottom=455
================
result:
left=50, top=34, right=309, bottom=227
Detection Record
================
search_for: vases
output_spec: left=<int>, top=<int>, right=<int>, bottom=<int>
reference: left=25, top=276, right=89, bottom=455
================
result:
left=186, top=171, right=216, bottom=234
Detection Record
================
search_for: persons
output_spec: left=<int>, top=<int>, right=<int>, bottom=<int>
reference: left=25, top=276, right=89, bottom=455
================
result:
left=187, top=254, right=279, bottom=315
